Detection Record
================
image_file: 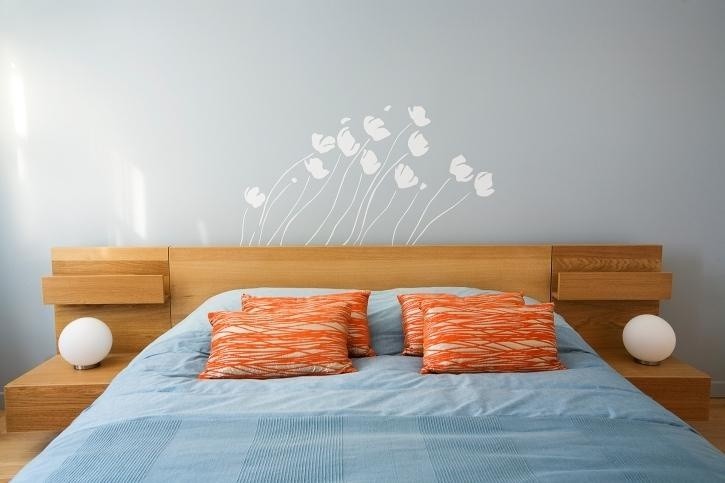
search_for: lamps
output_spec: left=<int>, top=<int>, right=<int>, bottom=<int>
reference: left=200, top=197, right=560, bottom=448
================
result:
left=57, top=317, right=113, bottom=370
left=622, top=312, right=677, bottom=365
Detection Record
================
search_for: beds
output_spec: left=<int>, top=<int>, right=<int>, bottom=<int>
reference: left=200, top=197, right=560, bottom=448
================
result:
left=3, top=285, right=725, bottom=483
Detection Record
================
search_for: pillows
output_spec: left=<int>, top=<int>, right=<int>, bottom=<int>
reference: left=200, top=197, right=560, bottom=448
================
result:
left=396, top=290, right=526, bottom=357
left=419, top=302, right=566, bottom=374
left=240, top=290, right=376, bottom=358
left=199, top=301, right=358, bottom=379
